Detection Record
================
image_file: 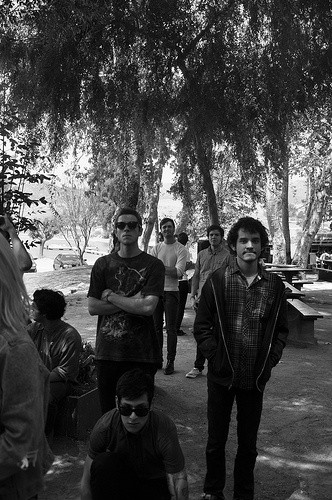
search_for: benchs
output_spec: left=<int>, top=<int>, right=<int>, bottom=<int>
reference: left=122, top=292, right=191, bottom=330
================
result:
left=317, top=268, right=332, bottom=282
left=282, top=277, right=313, bottom=284
left=287, top=299, right=324, bottom=344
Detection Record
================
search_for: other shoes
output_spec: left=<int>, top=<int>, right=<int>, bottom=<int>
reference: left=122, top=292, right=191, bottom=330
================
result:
left=165, top=363, right=174, bottom=375
left=163, top=326, right=167, bottom=329
left=177, top=329, right=186, bottom=336
left=200, top=492, right=225, bottom=500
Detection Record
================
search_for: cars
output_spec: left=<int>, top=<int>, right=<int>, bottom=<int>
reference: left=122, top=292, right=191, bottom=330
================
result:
left=52, top=253, right=88, bottom=272
left=23, top=251, right=38, bottom=273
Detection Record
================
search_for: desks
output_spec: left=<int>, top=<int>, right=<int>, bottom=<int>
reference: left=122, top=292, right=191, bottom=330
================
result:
left=264, top=263, right=313, bottom=284
left=283, top=281, right=305, bottom=295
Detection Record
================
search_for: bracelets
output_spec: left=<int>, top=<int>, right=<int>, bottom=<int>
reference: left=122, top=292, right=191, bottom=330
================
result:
left=102, top=291, right=112, bottom=303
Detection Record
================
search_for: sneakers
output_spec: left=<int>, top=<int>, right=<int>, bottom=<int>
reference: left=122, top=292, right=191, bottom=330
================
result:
left=186, top=368, right=202, bottom=378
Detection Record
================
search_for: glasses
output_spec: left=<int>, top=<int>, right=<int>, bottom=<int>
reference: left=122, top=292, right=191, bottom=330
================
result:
left=115, top=222, right=139, bottom=230
left=118, top=407, right=150, bottom=418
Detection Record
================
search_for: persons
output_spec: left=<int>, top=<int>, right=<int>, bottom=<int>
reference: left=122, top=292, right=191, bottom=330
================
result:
left=87, top=209, right=166, bottom=416
left=193, top=217, right=290, bottom=500
left=0, top=213, right=81, bottom=500
left=81, top=371, right=188, bottom=500
left=185, top=225, right=230, bottom=379
left=150, top=218, right=192, bottom=376
